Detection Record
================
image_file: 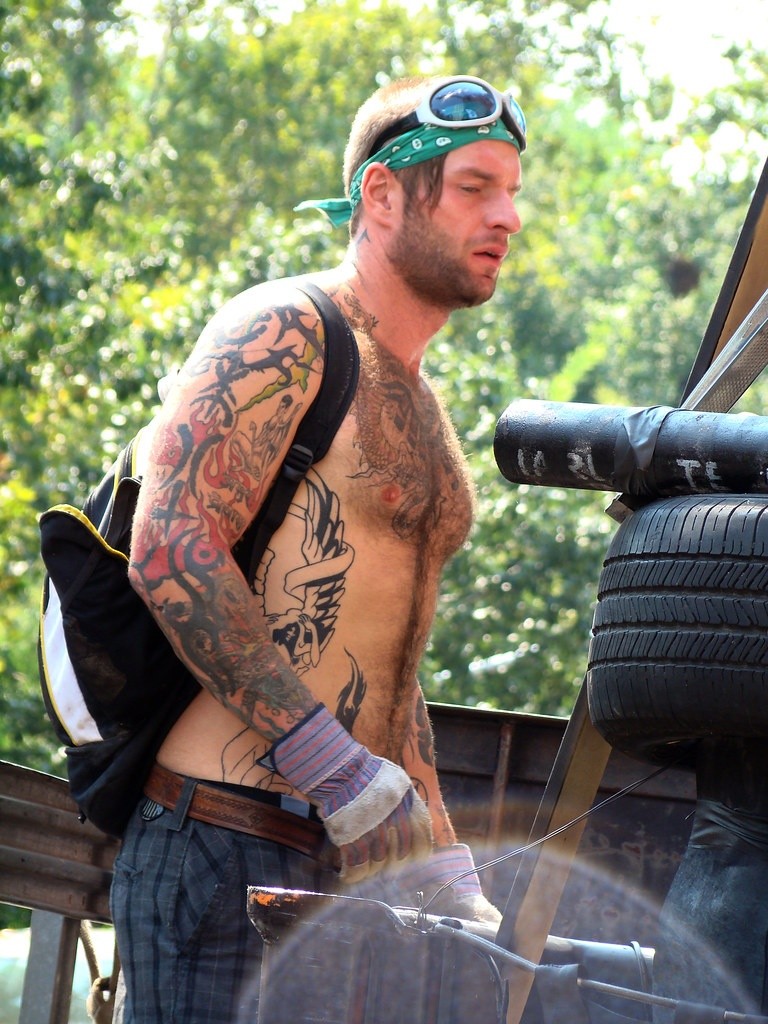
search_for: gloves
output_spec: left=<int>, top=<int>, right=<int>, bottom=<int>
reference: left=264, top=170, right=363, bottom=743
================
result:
left=397, top=843, right=503, bottom=923
left=253, top=703, right=434, bottom=883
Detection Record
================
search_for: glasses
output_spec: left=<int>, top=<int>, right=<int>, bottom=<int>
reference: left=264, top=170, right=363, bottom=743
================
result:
left=365, top=75, right=527, bottom=160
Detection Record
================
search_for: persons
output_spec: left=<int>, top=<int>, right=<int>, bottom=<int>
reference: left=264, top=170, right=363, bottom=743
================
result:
left=110, top=76, right=526, bottom=1024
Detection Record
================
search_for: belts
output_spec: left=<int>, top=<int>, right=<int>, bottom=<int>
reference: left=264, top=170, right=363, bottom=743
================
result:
left=143, top=764, right=342, bottom=870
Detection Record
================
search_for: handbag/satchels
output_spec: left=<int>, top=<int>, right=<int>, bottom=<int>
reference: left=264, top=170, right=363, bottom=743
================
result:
left=37, top=279, right=358, bottom=837
